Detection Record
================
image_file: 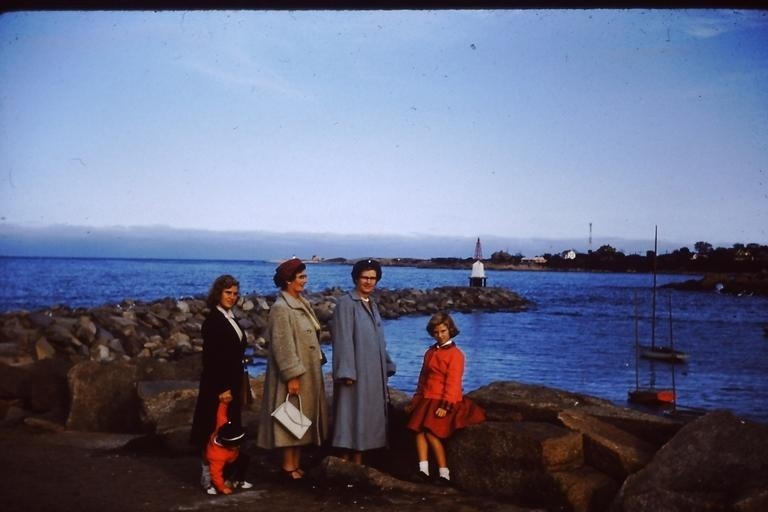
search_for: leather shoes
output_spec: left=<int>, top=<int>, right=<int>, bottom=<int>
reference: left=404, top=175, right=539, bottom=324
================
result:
left=409, top=471, right=457, bottom=486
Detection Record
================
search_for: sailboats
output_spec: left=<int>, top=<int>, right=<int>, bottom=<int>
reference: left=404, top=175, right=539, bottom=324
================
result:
left=634, top=224, right=691, bottom=361
left=627, top=288, right=675, bottom=404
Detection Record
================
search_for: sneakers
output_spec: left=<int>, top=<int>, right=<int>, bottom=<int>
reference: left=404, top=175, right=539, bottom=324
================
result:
left=206, top=485, right=217, bottom=494
left=236, top=481, right=254, bottom=488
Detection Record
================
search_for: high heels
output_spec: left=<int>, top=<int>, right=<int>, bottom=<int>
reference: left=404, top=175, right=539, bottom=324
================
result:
left=281, top=465, right=308, bottom=480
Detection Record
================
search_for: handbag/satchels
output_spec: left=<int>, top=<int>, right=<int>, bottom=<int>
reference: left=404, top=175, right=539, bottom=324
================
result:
left=241, top=370, right=256, bottom=407
left=271, top=401, right=312, bottom=440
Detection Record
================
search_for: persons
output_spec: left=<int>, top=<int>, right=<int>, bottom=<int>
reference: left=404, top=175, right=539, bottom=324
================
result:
left=253, top=258, right=331, bottom=488
left=191, top=274, right=254, bottom=480
left=199, top=393, right=253, bottom=495
left=402, top=310, right=486, bottom=485
left=328, top=256, right=397, bottom=466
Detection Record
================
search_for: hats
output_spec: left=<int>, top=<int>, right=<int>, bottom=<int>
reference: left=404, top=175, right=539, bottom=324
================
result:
left=351, top=258, right=381, bottom=285
left=274, top=258, right=306, bottom=287
left=217, top=421, right=249, bottom=447
